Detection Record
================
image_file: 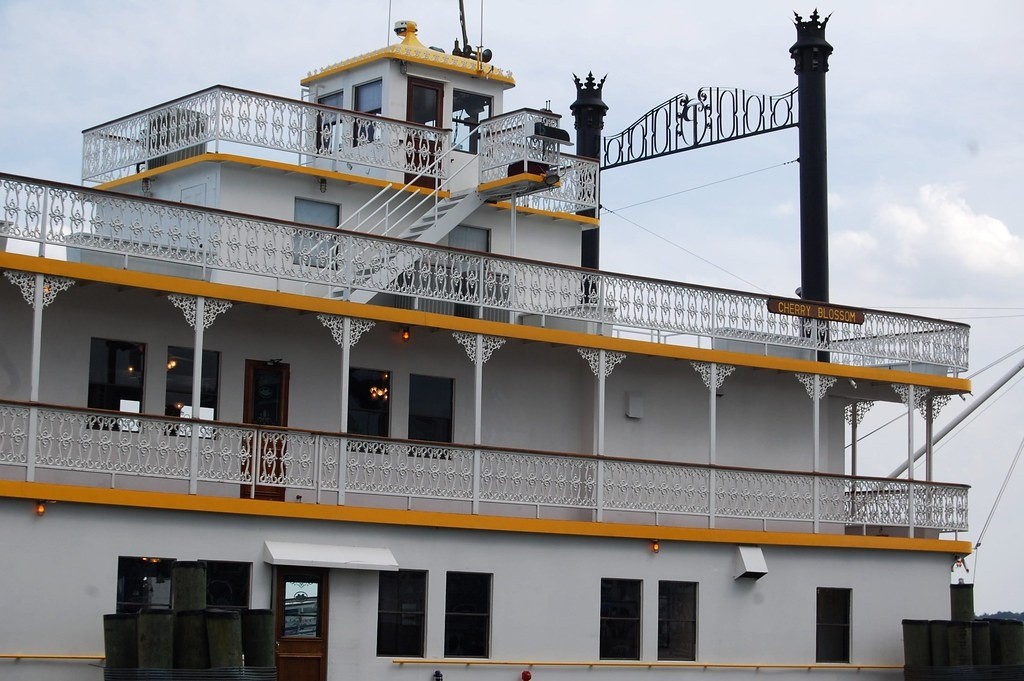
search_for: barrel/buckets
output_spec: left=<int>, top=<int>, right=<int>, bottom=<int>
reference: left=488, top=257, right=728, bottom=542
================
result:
left=241, top=609, right=276, bottom=667
left=950, top=584, right=974, bottom=621
left=104, top=613, right=138, bottom=668
left=174, top=609, right=210, bottom=670
left=206, top=608, right=244, bottom=668
left=137, top=608, right=176, bottom=670
left=171, top=560, right=207, bottom=610
left=902, top=618, right=1024, bottom=669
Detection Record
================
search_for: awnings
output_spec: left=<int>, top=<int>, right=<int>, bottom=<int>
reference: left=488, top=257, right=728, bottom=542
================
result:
left=262, top=540, right=399, bottom=572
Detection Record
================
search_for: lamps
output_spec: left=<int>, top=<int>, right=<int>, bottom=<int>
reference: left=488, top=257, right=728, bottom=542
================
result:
left=141, top=177, right=154, bottom=193
left=544, top=169, right=559, bottom=186
left=400, top=61, right=407, bottom=74
left=318, top=178, right=326, bottom=193
